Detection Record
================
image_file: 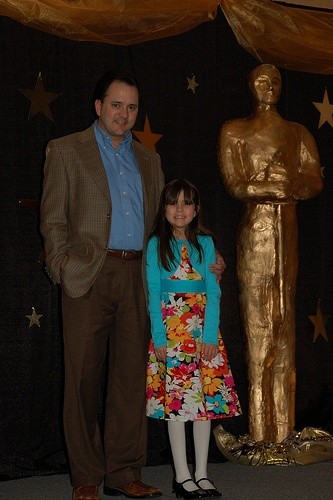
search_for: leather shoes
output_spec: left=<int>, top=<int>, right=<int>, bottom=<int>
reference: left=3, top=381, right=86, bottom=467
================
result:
left=72, top=484, right=101, bottom=500
left=194, top=477, right=222, bottom=500
left=103, top=479, right=162, bottom=499
left=173, top=478, right=213, bottom=500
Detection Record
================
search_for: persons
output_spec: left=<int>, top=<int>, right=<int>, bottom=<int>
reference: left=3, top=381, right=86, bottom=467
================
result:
left=145, top=177, right=243, bottom=500
left=39, top=71, right=226, bottom=500
left=216, top=63, right=323, bottom=445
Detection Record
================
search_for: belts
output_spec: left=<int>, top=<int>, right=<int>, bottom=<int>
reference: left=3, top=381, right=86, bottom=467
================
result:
left=107, top=250, right=143, bottom=261
